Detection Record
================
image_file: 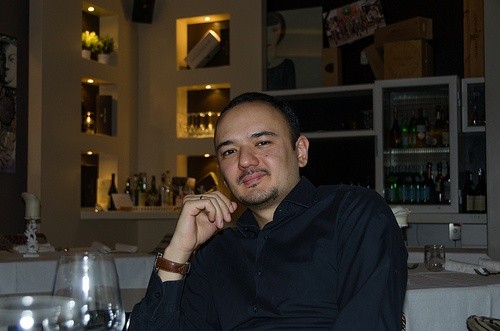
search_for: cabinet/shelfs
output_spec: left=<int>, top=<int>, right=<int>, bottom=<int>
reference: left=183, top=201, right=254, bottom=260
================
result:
left=374, top=75, right=459, bottom=214
left=28, top=0, right=262, bottom=252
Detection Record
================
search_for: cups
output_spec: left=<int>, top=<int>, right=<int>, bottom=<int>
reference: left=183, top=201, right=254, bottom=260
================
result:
left=424, top=243, right=445, bottom=272
left=49, top=251, right=126, bottom=331
left=188, top=112, right=221, bottom=135
left=0, top=294, right=83, bottom=331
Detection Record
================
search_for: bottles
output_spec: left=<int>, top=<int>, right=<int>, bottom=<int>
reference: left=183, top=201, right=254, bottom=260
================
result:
left=107, top=173, right=116, bottom=211
left=83, top=112, right=95, bottom=134
left=175, top=186, right=184, bottom=207
left=124, top=178, right=133, bottom=202
left=135, top=177, right=147, bottom=207
left=459, top=168, right=487, bottom=214
left=147, top=176, right=160, bottom=206
left=390, top=106, right=448, bottom=148
left=385, top=161, right=451, bottom=205
left=160, top=170, right=173, bottom=206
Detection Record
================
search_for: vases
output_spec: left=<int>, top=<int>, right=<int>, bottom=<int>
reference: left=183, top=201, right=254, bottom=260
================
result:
left=82, top=50, right=92, bottom=60
left=97, top=54, right=108, bottom=64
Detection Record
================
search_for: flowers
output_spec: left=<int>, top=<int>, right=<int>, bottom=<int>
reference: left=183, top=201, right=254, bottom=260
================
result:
left=81, top=30, right=98, bottom=50
left=96, top=33, right=115, bottom=54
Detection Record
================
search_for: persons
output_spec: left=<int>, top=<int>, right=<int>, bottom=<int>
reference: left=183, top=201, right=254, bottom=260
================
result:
left=126, top=92, right=410, bottom=331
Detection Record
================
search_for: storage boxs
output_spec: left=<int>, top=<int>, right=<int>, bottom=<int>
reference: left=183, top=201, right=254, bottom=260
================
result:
left=318, top=17, right=432, bottom=86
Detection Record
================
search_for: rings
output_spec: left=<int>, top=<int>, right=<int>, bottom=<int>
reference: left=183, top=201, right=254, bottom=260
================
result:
left=199, top=195, right=202, bottom=198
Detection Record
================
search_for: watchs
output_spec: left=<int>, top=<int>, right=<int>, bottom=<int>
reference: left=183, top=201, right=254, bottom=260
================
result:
left=154, top=252, right=192, bottom=276
